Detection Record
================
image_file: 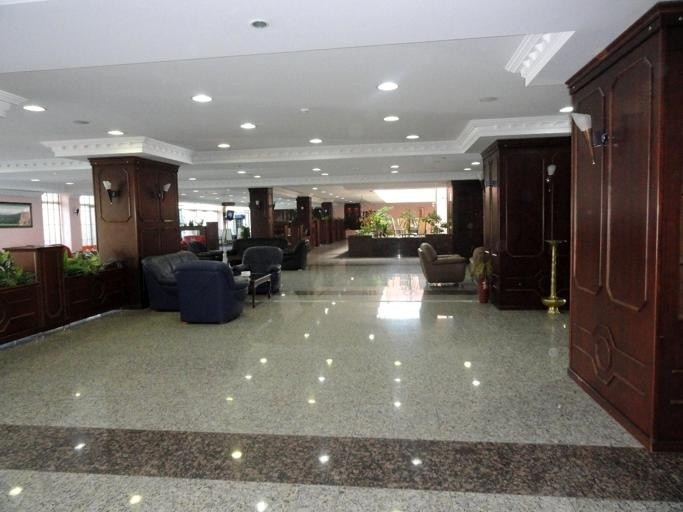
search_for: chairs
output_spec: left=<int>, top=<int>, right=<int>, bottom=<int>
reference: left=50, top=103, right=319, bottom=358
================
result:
left=187, top=241, right=223, bottom=262
left=174, top=260, right=250, bottom=324
left=417, top=242, right=467, bottom=286
left=233, top=245, right=283, bottom=295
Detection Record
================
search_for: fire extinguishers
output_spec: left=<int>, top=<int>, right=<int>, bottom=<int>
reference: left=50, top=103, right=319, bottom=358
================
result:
left=478, top=272, right=489, bottom=304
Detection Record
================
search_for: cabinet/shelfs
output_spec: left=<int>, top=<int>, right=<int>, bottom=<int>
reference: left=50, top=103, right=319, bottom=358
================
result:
left=564, top=0, right=682, bottom=454
left=480, top=134, right=571, bottom=310
left=446, top=179, right=483, bottom=264
left=180, top=222, right=219, bottom=251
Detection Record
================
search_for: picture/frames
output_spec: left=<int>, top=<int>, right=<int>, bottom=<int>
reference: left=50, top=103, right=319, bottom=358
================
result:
left=0, top=202, right=32, bottom=228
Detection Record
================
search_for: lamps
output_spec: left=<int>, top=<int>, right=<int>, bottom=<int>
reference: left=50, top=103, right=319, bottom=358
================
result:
left=157, top=183, right=170, bottom=202
left=571, top=112, right=608, bottom=165
left=103, top=180, right=118, bottom=205
left=544, top=165, right=556, bottom=183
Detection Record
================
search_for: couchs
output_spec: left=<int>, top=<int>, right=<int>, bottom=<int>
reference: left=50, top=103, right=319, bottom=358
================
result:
left=227, top=236, right=307, bottom=271
left=141, top=251, right=199, bottom=312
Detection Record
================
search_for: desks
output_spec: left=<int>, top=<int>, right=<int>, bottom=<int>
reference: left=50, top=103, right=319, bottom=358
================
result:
left=232, top=271, right=271, bottom=308
